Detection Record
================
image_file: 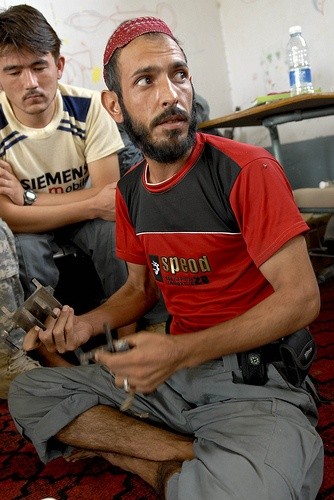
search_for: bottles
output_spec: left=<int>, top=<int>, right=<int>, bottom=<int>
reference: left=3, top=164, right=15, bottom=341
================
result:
left=288, top=26, right=314, bottom=97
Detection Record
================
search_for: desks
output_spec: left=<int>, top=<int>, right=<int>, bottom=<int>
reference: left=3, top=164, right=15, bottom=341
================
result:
left=195, top=90, right=334, bottom=167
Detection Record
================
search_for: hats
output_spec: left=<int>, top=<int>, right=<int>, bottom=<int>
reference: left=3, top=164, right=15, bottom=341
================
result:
left=103, top=16, right=175, bottom=83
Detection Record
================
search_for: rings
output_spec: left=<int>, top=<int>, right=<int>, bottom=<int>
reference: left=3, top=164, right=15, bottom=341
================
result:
left=122, top=376, right=130, bottom=393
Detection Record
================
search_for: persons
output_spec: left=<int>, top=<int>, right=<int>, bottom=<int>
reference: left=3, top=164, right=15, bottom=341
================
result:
left=0, top=9, right=137, bottom=366
left=8, top=17, right=324, bottom=500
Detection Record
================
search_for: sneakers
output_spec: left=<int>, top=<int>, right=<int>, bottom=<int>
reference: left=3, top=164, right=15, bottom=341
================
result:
left=146, top=322, right=168, bottom=334
left=0, top=347, right=45, bottom=399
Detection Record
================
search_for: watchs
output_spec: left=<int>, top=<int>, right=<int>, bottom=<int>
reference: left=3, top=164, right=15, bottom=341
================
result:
left=23, top=189, right=37, bottom=206
